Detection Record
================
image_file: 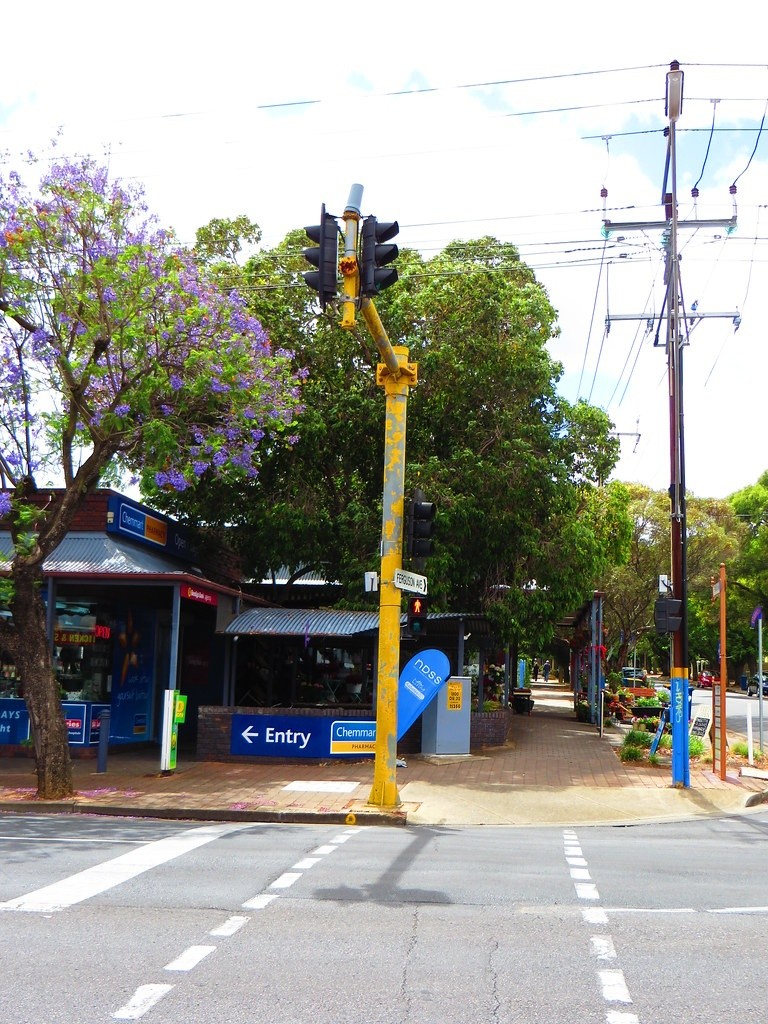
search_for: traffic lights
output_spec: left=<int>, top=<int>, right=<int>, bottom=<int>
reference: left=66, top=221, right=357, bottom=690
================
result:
left=665, top=598, right=684, bottom=633
left=303, top=203, right=338, bottom=311
left=408, top=500, right=436, bottom=557
left=361, top=215, right=397, bottom=297
left=408, top=596, right=428, bottom=636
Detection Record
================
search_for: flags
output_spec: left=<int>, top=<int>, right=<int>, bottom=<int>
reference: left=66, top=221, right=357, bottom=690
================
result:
left=750, top=607, right=761, bottom=628
left=717, top=641, right=720, bottom=665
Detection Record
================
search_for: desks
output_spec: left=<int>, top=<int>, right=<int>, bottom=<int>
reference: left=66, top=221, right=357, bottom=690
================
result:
left=513, top=693, right=532, bottom=716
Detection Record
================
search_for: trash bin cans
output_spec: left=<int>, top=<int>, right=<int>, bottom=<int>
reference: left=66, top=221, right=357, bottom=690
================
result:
left=623, top=678, right=643, bottom=698
left=663, top=684, right=694, bottom=720
left=740, top=674, right=747, bottom=691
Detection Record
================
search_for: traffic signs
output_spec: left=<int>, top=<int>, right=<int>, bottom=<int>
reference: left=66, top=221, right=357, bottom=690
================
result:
left=394, top=568, right=428, bottom=596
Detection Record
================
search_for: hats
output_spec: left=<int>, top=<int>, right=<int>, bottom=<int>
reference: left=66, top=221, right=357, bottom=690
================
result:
left=546, top=660, right=549, bottom=662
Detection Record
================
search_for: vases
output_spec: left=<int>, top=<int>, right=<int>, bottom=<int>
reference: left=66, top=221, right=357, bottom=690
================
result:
left=345, top=683, right=363, bottom=693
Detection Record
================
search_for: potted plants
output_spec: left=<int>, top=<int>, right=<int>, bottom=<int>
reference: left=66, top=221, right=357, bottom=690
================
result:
left=605, top=690, right=672, bottom=733
left=22, top=736, right=37, bottom=759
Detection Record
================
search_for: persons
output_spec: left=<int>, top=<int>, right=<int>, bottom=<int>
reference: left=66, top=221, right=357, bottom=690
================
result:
left=532, top=664, right=539, bottom=681
left=542, top=660, right=551, bottom=682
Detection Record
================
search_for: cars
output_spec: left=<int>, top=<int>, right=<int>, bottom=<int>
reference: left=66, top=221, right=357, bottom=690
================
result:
left=697, top=670, right=720, bottom=688
left=747, top=671, right=768, bottom=698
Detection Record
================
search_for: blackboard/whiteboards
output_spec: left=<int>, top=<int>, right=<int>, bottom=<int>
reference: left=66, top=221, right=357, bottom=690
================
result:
left=689, top=716, right=729, bottom=746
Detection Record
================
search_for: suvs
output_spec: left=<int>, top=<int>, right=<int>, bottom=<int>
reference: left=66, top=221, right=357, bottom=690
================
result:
left=621, top=667, right=647, bottom=686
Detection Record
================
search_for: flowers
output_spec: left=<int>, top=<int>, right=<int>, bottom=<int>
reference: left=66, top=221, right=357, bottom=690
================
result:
left=337, top=666, right=370, bottom=685
